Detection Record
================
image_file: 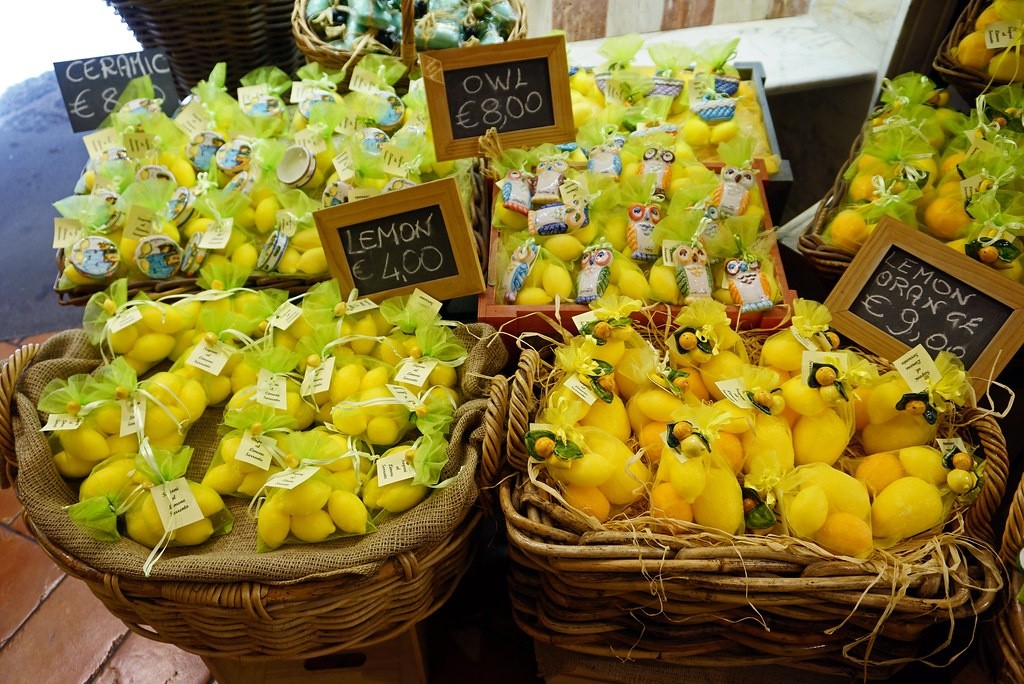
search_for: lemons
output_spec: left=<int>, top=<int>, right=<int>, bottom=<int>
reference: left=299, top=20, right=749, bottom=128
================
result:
left=49, top=75, right=1021, bottom=552
left=957, top=0, right=1024, bottom=82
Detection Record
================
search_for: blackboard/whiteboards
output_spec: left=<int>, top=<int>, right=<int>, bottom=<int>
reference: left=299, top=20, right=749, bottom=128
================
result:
left=311, top=177, right=486, bottom=308
left=820, top=214, right=1024, bottom=407
left=53, top=48, right=186, bottom=134
left=419, top=35, right=577, bottom=162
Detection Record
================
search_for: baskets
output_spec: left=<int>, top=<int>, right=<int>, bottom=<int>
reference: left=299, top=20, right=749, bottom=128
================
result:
left=291, top=0, right=527, bottom=98
left=503, top=335, right=1006, bottom=680
left=0, top=322, right=508, bottom=664
left=51, top=146, right=486, bottom=309
left=102, top=0, right=305, bottom=102
left=931, top=0, right=1016, bottom=109
left=990, top=469, right=1024, bottom=684
left=799, top=103, right=884, bottom=273
